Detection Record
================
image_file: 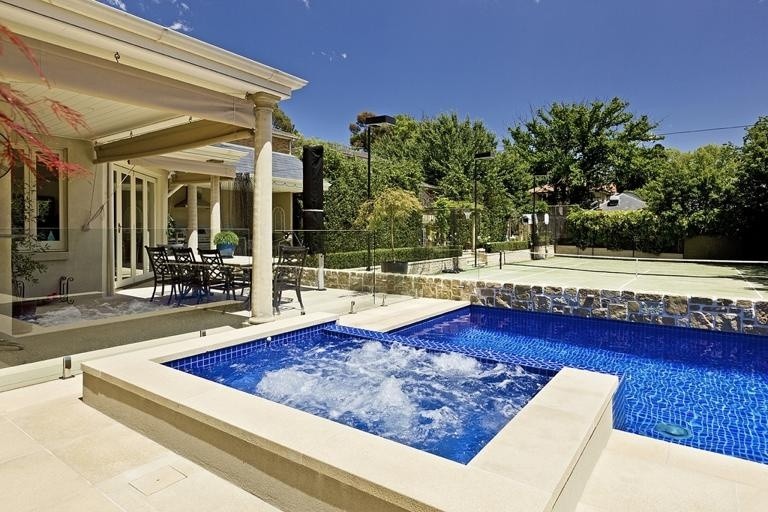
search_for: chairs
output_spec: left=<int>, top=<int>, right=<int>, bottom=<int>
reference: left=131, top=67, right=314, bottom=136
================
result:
left=144, top=245, right=310, bottom=316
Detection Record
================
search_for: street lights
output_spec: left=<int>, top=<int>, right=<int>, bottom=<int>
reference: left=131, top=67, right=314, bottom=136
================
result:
left=474, top=152, right=494, bottom=267
left=532, top=171, right=547, bottom=259
left=365, top=115, right=396, bottom=271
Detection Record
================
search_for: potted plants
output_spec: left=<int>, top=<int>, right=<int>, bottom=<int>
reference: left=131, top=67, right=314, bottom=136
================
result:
left=213, top=230, right=240, bottom=258
